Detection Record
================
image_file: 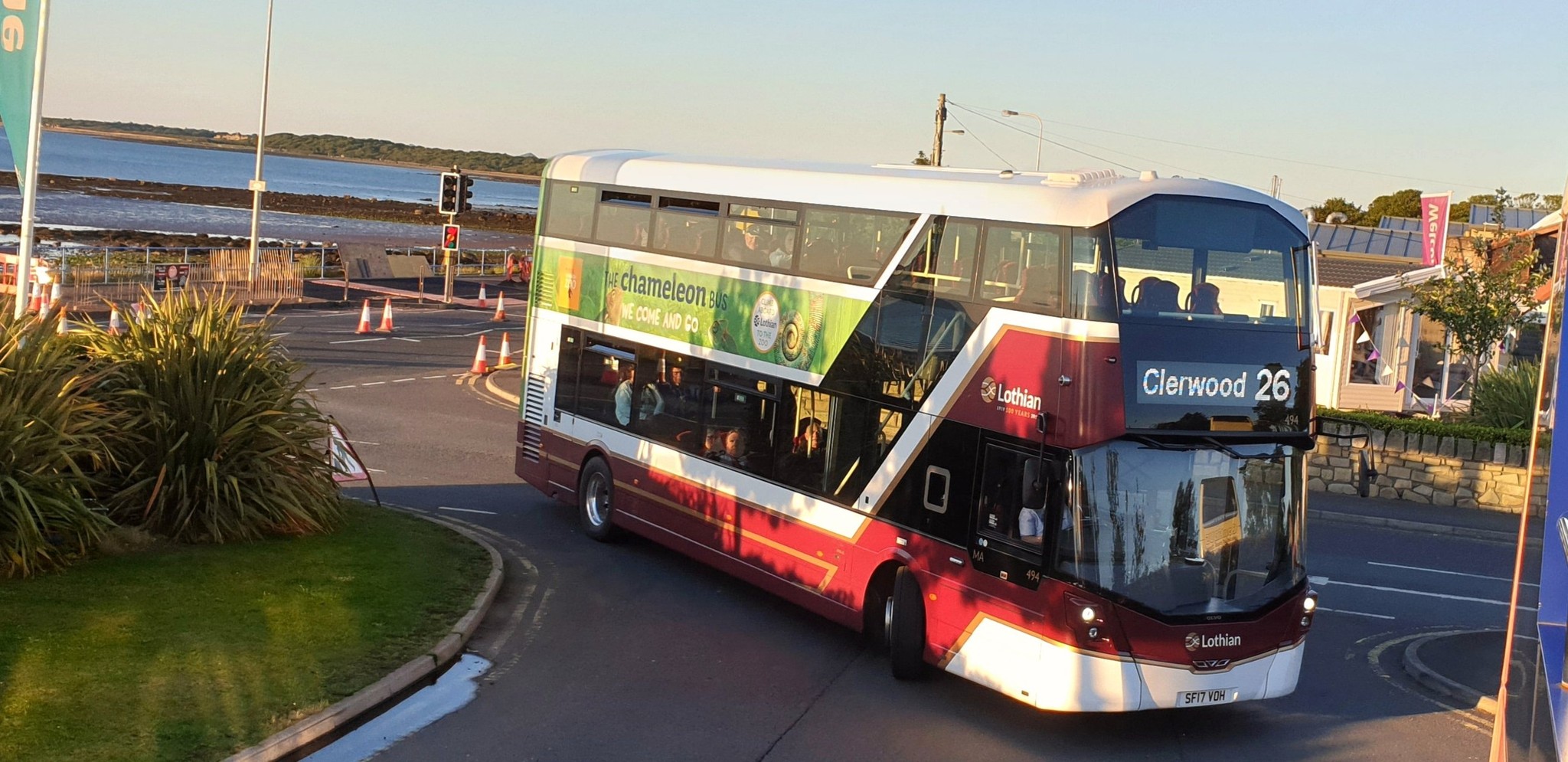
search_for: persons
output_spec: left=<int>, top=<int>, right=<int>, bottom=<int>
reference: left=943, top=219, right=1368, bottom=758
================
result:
left=641, top=213, right=797, bottom=268
left=614, top=364, right=825, bottom=471
left=1019, top=502, right=1073, bottom=545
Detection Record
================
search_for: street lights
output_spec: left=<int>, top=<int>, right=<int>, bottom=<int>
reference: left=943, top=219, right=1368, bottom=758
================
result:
left=1001, top=110, right=1043, bottom=172
left=930, top=128, right=965, bottom=165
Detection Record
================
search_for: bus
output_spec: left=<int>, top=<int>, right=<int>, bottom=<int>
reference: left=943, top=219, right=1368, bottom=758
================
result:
left=509, top=150, right=1378, bottom=718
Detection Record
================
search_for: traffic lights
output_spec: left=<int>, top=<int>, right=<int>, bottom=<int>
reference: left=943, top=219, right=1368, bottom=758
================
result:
left=438, top=172, right=463, bottom=215
left=441, top=224, right=462, bottom=250
left=458, top=173, right=474, bottom=214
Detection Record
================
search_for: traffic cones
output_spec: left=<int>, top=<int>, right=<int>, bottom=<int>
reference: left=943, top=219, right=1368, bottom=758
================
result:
left=133, top=301, right=146, bottom=325
left=469, top=335, right=491, bottom=375
left=497, top=333, right=515, bottom=366
left=27, top=276, right=43, bottom=317
left=48, top=275, right=63, bottom=310
left=477, top=282, right=489, bottom=309
left=490, top=290, right=512, bottom=322
left=355, top=299, right=376, bottom=334
left=38, top=283, right=50, bottom=325
left=53, top=306, right=68, bottom=336
left=108, top=302, right=120, bottom=336
left=375, top=296, right=399, bottom=333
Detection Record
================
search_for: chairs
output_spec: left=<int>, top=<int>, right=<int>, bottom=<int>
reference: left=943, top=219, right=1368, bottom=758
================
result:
left=565, top=212, right=1220, bottom=315
left=577, top=366, right=823, bottom=459
